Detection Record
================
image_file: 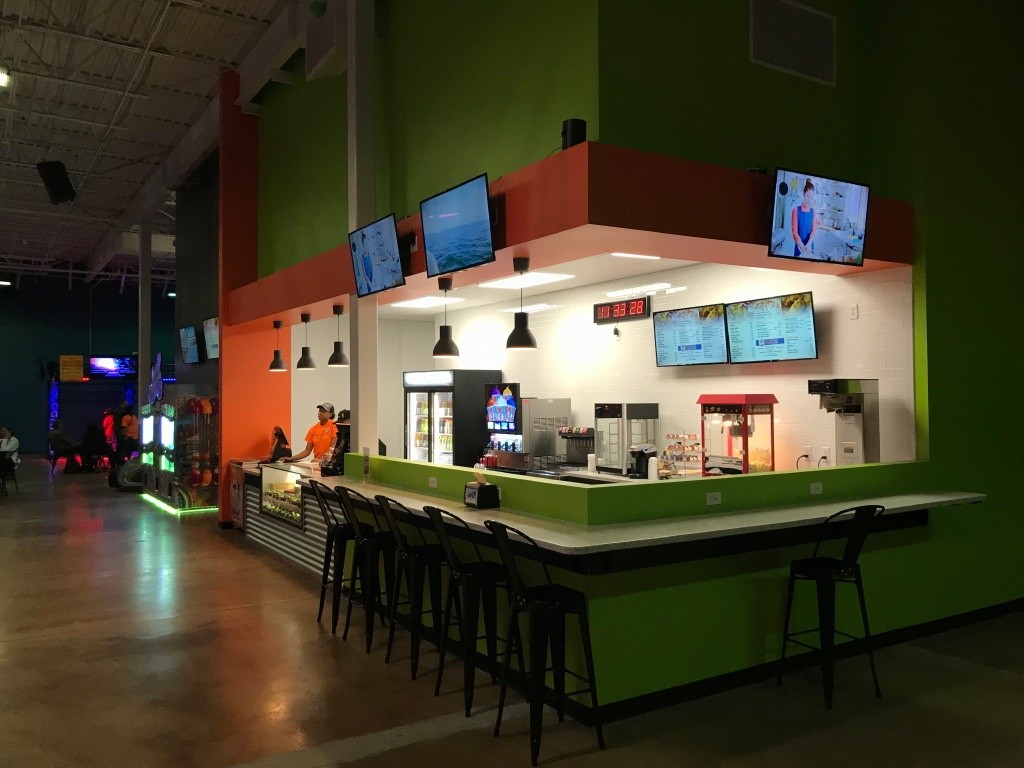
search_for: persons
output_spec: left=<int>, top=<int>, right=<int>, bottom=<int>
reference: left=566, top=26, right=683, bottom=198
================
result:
left=285, top=403, right=338, bottom=466
left=0, top=426, right=18, bottom=464
left=82, top=400, right=138, bottom=487
left=48, top=420, right=73, bottom=452
left=333, top=409, right=350, bottom=424
left=361, top=234, right=374, bottom=293
left=792, top=178, right=817, bottom=257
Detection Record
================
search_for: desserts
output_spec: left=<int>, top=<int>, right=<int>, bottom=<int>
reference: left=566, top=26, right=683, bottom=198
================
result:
left=665, top=433, right=700, bottom=462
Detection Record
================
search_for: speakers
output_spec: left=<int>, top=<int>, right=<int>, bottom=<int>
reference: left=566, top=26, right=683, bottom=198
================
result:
left=562, top=118, right=587, bottom=149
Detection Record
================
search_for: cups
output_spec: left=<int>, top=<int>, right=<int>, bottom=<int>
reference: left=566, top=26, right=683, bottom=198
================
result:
left=587, top=454, right=596, bottom=472
left=648, top=456, right=658, bottom=480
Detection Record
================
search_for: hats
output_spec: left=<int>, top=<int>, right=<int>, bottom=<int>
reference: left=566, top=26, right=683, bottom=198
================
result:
left=317, top=403, right=334, bottom=413
left=334, top=409, right=350, bottom=425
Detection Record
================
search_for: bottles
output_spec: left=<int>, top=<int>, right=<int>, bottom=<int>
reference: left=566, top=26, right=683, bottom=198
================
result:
left=415, top=399, right=453, bottom=452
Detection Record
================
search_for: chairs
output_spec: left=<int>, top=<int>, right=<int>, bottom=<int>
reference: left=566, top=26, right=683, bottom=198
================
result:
left=0, top=448, right=20, bottom=496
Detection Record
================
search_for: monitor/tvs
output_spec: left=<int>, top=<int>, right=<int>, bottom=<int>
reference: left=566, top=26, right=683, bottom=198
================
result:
left=420, top=171, right=495, bottom=278
left=767, top=168, right=871, bottom=267
left=178, top=324, right=201, bottom=364
left=348, top=212, right=405, bottom=298
left=725, top=291, right=819, bottom=364
left=202, top=316, right=220, bottom=359
left=484, top=383, right=521, bottom=434
left=653, top=303, right=729, bottom=366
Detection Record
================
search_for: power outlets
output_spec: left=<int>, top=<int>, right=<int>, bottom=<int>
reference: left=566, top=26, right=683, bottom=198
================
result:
left=803, top=446, right=811, bottom=461
left=822, top=447, right=830, bottom=462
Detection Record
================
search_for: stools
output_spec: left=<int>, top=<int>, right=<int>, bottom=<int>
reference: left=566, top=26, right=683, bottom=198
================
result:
left=307, top=480, right=607, bottom=767
left=777, top=505, right=885, bottom=708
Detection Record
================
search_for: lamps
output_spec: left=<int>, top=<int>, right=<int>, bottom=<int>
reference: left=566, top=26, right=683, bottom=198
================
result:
left=296, top=312, right=316, bottom=371
left=507, top=257, right=537, bottom=350
left=431, top=275, right=458, bottom=358
left=329, top=302, right=350, bottom=366
left=269, top=321, right=289, bottom=373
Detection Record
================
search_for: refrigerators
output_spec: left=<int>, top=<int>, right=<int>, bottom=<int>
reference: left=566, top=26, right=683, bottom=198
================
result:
left=404, top=370, right=503, bottom=471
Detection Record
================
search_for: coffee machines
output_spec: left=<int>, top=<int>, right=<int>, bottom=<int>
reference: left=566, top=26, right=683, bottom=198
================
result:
left=628, top=443, right=657, bottom=480
